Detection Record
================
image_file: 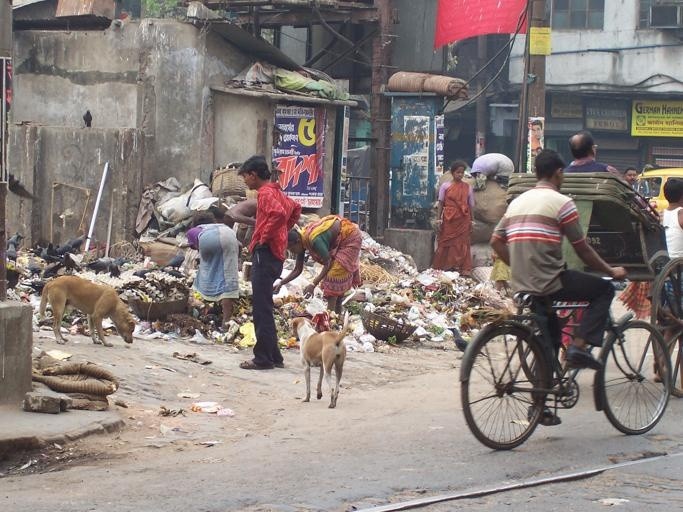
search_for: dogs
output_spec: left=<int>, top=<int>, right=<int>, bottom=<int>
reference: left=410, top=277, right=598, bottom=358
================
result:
left=288, top=316, right=360, bottom=410
left=39, top=274, right=141, bottom=348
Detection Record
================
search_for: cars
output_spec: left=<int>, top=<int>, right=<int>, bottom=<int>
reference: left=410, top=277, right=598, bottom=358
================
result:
left=630, top=167, right=682, bottom=216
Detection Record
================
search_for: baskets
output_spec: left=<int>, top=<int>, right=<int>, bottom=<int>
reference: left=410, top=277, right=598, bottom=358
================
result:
left=212, top=163, right=248, bottom=197
left=359, top=309, right=417, bottom=343
left=128, top=282, right=188, bottom=321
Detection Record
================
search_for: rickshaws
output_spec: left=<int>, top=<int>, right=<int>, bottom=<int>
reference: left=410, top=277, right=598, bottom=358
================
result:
left=506, top=170, right=682, bottom=399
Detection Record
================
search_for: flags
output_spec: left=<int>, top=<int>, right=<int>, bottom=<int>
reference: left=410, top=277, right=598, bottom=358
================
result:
left=430, top=0, right=527, bottom=51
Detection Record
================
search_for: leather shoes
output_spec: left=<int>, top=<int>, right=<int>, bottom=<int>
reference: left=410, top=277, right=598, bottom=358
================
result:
left=528, top=405, right=561, bottom=426
left=567, top=344, right=602, bottom=371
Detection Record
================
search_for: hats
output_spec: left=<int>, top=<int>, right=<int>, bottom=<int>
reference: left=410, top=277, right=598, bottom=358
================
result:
left=238, top=156, right=265, bottom=174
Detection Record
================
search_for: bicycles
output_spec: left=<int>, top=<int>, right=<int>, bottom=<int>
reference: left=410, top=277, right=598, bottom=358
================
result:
left=457, top=271, right=672, bottom=451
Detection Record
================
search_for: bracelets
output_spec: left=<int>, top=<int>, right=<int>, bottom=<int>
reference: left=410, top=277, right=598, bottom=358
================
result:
left=434, top=218, right=443, bottom=223
left=471, top=220, right=475, bottom=225
left=309, top=282, right=316, bottom=288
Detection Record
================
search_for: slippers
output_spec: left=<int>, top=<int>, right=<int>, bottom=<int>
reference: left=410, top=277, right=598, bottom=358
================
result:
left=240, top=360, right=284, bottom=369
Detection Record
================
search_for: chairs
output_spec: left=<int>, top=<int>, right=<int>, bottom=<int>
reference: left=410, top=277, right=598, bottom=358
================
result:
left=503, top=171, right=668, bottom=276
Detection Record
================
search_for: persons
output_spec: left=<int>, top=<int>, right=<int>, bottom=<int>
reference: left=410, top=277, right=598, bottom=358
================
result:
left=238, top=154, right=301, bottom=371
left=530, top=120, right=545, bottom=155
left=210, top=200, right=257, bottom=265
left=555, top=129, right=682, bottom=382
left=187, top=212, right=239, bottom=335
left=433, top=159, right=476, bottom=281
left=482, top=150, right=626, bottom=430
left=272, top=215, right=363, bottom=322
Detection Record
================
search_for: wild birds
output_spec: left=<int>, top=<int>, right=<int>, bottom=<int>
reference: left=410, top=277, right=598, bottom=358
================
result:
left=5, top=232, right=186, bottom=296
